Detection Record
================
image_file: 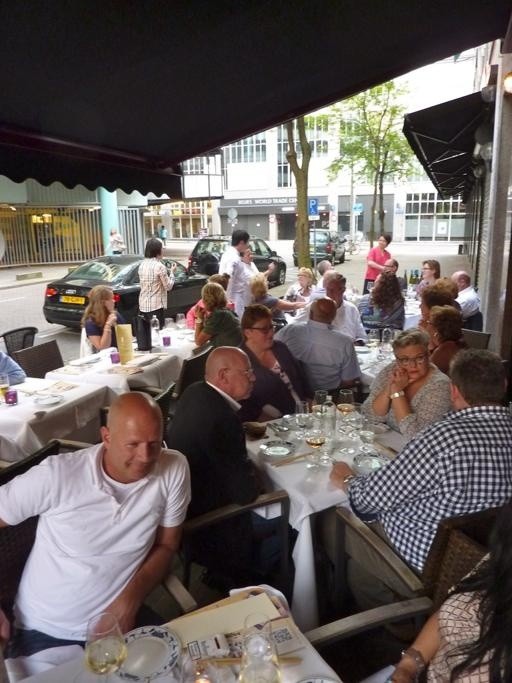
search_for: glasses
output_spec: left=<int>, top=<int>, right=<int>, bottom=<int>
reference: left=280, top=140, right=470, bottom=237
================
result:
left=248, top=322, right=275, bottom=334
left=395, top=353, right=429, bottom=365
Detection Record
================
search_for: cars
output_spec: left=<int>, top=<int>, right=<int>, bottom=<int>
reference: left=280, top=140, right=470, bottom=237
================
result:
left=290, top=229, right=349, bottom=266
left=43, top=252, right=216, bottom=334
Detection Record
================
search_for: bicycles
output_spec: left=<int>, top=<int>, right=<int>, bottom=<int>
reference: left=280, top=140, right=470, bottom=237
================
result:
left=345, top=236, right=361, bottom=255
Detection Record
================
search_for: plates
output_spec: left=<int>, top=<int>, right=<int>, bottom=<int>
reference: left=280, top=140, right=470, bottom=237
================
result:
left=20, top=324, right=195, bottom=405
left=116, top=623, right=182, bottom=682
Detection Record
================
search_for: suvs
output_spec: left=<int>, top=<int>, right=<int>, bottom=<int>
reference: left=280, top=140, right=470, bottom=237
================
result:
left=183, top=234, right=287, bottom=288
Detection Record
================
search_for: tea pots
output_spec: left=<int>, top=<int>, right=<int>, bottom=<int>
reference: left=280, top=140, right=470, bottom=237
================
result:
left=133, top=309, right=151, bottom=349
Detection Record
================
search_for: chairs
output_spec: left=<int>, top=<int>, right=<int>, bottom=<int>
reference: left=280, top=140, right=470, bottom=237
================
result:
left=47, top=384, right=176, bottom=452
left=329, top=505, right=512, bottom=612
left=300, top=526, right=492, bottom=680
left=461, top=328, right=491, bottom=351
left=10, top=339, right=66, bottom=381
left=1, top=440, right=57, bottom=485
left=2, top=516, right=199, bottom=662
left=2, top=325, right=38, bottom=356
left=183, top=488, right=293, bottom=606
left=142, top=345, right=217, bottom=404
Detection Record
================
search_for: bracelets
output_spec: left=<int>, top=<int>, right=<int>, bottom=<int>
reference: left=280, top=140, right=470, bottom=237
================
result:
left=343, top=474, right=354, bottom=483
left=390, top=391, right=404, bottom=399
left=400, top=647, right=426, bottom=680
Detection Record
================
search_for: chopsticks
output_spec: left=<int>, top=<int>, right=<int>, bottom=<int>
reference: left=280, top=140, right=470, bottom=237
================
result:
left=199, top=656, right=303, bottom=666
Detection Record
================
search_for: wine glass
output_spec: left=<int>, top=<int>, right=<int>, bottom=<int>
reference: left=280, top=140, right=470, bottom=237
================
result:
left=367, top=281, right=375, bottom=298
left=165, top=317, right=175, bottom=336
left=259, top=384, right=398, bottom=476
left=402, top=286, right=421, bottom=315
left=86, top=612, right=129, bottom=682
left=353, top=324, right=404, bottom=372
left=343, top=279, right=360, bottom=304
left=176, top=313, right=186, bottom=338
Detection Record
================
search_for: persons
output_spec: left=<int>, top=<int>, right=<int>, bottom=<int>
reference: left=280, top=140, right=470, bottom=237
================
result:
left=219, top=229, right=259, bottom=313
left=160, top=225, right=168, bottom=247
left=357, top=516, right=511, bottom=682
left=329, top=349, right=512, bottom=610
left=0, top=351, right=25, bottom=387
left=108, top=227, right=124, bottom=254
left=357, top=258, right=480, bottom=375
left=363, top=233, right=392, bottom=296
left=138, top=237, right=177, bottom=331
left=81, top=284, right=124, bottom=351
left=188, top=258, right=369, bottom=422
left=361, top=327, right=454, bottom=440
left=169, top=346, right=283, bottom=586
left=1, top=392, right=192, bottom=660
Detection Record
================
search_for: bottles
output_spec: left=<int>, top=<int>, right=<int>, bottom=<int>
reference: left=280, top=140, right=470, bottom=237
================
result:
left=404, top=268, right=423, bottom=285
left=150, top=313, right=162, bottom=346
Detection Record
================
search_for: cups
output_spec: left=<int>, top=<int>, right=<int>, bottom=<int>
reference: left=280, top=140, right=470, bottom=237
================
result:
left=193, top=610, right=284, bottom=682
left=4, top=387, right=17, bottom=405
left=0, top=372, right=10, bottom=402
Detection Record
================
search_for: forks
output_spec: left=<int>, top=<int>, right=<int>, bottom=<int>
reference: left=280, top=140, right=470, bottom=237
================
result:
left=240, top=614, right=292, bottom=636
